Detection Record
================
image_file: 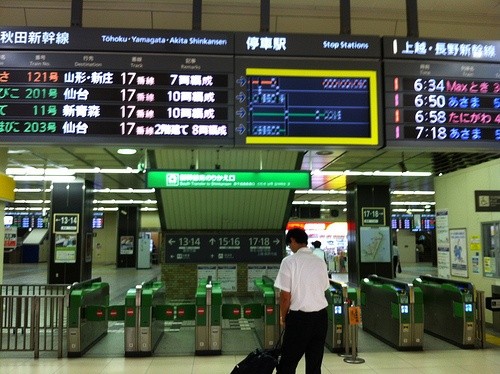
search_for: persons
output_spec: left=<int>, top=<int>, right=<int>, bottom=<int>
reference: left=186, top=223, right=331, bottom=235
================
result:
left=273, top=228, right=330, bottom=374
left=311, top=241, right=328, bottom=269
left=392, top=240, right=399, bottom=278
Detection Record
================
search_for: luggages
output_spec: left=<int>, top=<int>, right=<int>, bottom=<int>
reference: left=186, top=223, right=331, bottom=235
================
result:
left=230, top=327, right=285, bottom=374
